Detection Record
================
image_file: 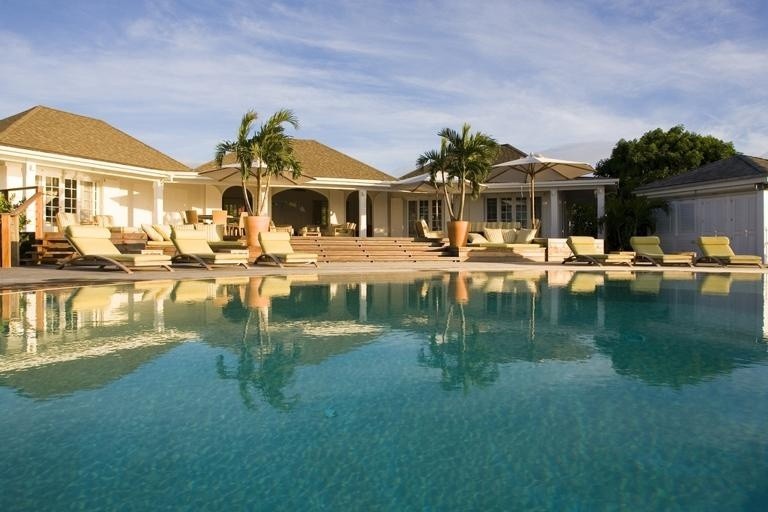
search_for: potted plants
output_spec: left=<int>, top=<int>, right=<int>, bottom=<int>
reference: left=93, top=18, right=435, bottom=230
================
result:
left=215, top=109, right=302, bottom=245
left=415, top=123, right=502, bottom=248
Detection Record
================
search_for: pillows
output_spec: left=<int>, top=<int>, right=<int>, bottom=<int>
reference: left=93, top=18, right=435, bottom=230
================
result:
left=468, top=227, right=537, bottom=245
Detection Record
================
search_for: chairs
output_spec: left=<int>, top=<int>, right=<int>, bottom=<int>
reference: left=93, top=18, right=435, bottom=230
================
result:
left=330, top=222, right=356, bottom=237
left=185, top=207, right=248, bottom=238
left=270, top=220, right=294, bottom=235
left=567, top=236, right=765, bottom=269
left=58, top=224, right=321, bottom=274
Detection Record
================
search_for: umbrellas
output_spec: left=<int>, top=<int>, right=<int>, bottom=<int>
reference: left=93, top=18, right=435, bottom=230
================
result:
left=508, top=293, right=599, bottom=362
left=479, top=152, right=597, bottom=228
left=390, top=169, right=487, bottom=231
